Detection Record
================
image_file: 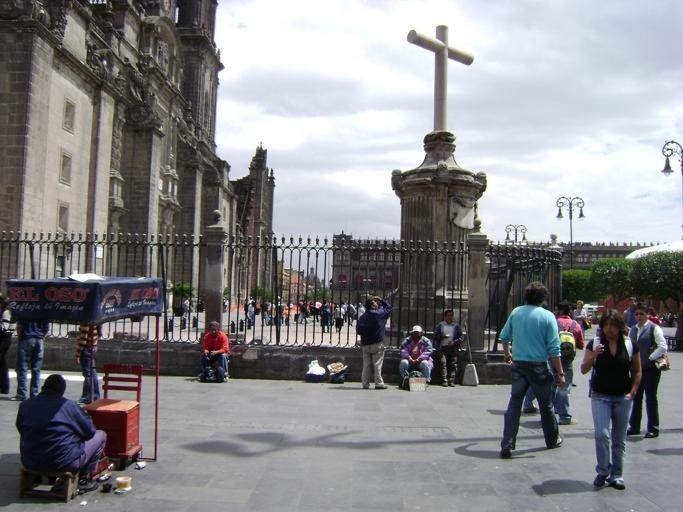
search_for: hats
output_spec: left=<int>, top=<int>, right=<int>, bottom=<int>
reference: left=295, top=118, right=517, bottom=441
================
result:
left=409, top=325, right=423, bottom=334
left=365, top=295, right=374, bottom=308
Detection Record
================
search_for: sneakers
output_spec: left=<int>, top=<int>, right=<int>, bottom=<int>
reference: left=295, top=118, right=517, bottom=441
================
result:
left=523, top=407, right=539, bottom=413
left=612, top=478, right=625, bottom=490
left=375, top=385, right=389, bottom=389
left=594, top=475, right=606, bottom=490
left=449, top=381, right=454, bottom=386
left=559, top=415, right=574, bottom=425
left=199, top=373, right=204, bottom=379
left=547, top=436, right=563, bottom=449
left=626, top=427, right=638, bottom=434
left=362, top=385, right=368, bottom=388
left=76, top=479, right=100, bottom=496
left=442, top=380, right=447, bottom=384
left=216, top=378, right=225, bottom=382
left=500, top=447, right=509, bottom=459
left=645, top=430, right=660, bottom=437
left=426, top=383, right=428, bottom=387
left=8, top=395, right=23, bottom=400
left=399, top=383, right=404, bottom=389
left=74, top=399, right=91, bottom=406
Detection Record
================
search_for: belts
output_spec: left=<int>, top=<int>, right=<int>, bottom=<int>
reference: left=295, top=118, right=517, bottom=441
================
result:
left=513, top=360, right=548, bottom=367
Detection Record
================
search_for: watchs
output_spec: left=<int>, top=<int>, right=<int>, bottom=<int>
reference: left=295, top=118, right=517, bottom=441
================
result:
left=630, top=390, right=637, bottom=398
left=558, top=372, right=565, bottom=376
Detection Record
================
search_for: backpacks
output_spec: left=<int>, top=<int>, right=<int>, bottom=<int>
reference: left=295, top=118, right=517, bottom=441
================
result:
left=555, top=319, right=576, bottom=360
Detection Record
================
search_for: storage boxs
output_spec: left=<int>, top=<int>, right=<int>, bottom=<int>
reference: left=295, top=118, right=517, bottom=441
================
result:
left=81, top=399, right=140, bottom=471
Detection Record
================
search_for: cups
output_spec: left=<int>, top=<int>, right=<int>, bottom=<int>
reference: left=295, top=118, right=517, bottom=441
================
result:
left=116, top=476, right=133, bottom=490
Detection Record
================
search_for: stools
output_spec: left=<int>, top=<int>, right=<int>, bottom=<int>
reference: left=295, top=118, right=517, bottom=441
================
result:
left=19, top=467, right=80, bottom=503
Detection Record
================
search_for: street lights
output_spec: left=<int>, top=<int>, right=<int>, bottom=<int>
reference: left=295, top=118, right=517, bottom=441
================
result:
left=661, top=141, right=682, bottom=177
left=505, top=225, right=526, bottom=242
left=557, top=196, right=584, bottom=266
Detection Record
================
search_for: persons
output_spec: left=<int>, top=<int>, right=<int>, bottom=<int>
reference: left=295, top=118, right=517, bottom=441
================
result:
left=657, top=306, right=680, bottom=328
left=16, top=375, right=107, bottom=495
left=199, top=320, right=230, bottom=383
left=184, top=297, right=191, bottom=320
left=580, top=306, right=642, bottom=490
left=16, top=321, right=50, bottom=401
left=499, top=281, right=567, bottom=460
left=244, top=295, right=365, bottom=333
left=626, top=305, right=668, bottom=438
left=0, top=291, right=16, bottom=399
left=435, top=309, right=465, bottom=387
left=623, top=297, right=640, bottom=335
left=397, top=324, right=433, bottom=391
left=646, top=306, right=661, bottom=327
left=225, top=298, right=228, bottom=312
left=574, top=298, right=587, bottom=340
left=356, top=295, right=393, bottom=390
left=551, top=299, right=584, bottom=426
left=523, top=381, right=538, bottom=415
left=74, top=321, right=101, bottom=408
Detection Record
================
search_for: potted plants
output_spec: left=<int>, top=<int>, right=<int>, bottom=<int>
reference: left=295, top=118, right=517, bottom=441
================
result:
left=223, top=287, right=231, bottom=314
left=250, top=286, right=274, bottom=315
left=170, top=279, right=198, bottom=317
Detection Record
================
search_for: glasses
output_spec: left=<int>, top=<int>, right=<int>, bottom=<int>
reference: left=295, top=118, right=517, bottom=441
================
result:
left=633, top=310, right=648, bottom=317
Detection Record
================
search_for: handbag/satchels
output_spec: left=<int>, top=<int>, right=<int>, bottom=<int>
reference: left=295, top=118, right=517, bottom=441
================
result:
left=402, top=372, right=424, bottom=390
left=329, top=365, right=350, bottom=384
left=304, top=371, right=327, bottom=383
left=649, top=323, right=671, bottom=372
left=204, top=358, right=216, bottom=383
left=582, top=314, right=591, bottom=329
